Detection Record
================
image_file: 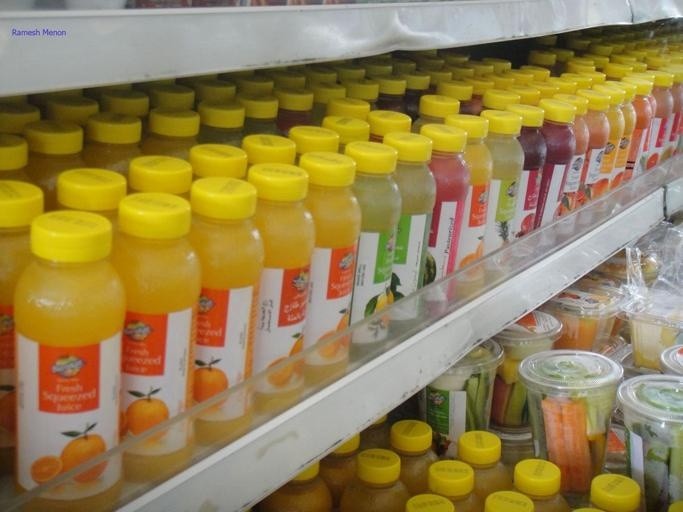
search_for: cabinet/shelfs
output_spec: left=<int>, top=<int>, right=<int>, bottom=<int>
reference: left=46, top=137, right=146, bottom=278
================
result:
left=0, top=1, right=683, bottom=512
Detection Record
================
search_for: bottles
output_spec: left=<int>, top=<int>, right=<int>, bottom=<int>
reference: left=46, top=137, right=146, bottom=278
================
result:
left=0, top=19, right=683, bottom=512
